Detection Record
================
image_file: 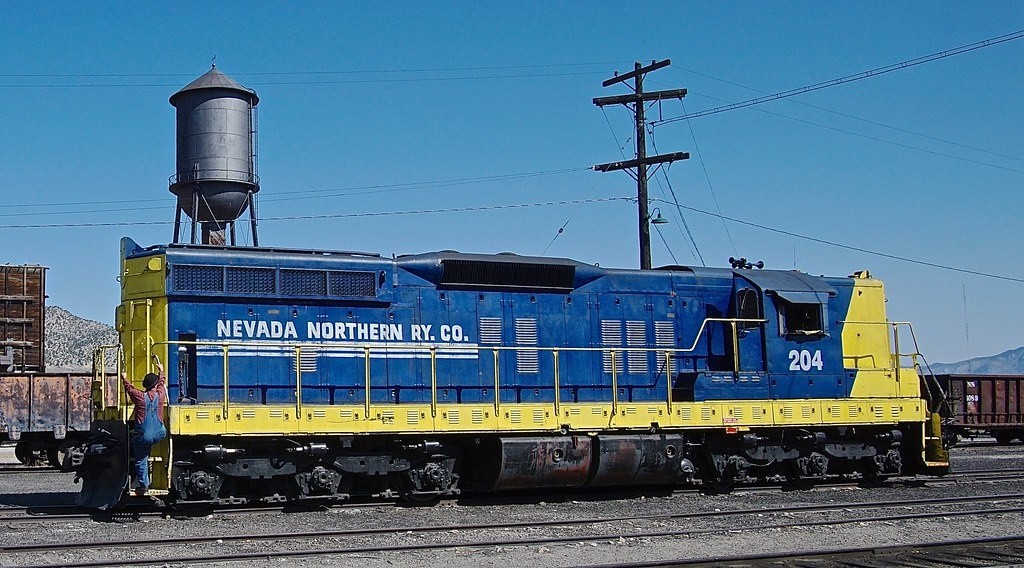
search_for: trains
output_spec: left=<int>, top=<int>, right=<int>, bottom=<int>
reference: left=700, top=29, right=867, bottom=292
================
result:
left=72, top=236, right=958, bottom=510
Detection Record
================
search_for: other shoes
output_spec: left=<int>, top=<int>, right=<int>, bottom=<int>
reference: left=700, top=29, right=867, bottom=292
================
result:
left=136, top=486, right=148, bottom=492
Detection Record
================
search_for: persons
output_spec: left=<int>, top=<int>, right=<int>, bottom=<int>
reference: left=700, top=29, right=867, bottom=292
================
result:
left=120, top=362, right=167, bottom=494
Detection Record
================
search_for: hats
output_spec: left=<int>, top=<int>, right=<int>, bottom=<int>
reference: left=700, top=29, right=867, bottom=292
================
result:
left=142, top=373, right=159, bottom=388
